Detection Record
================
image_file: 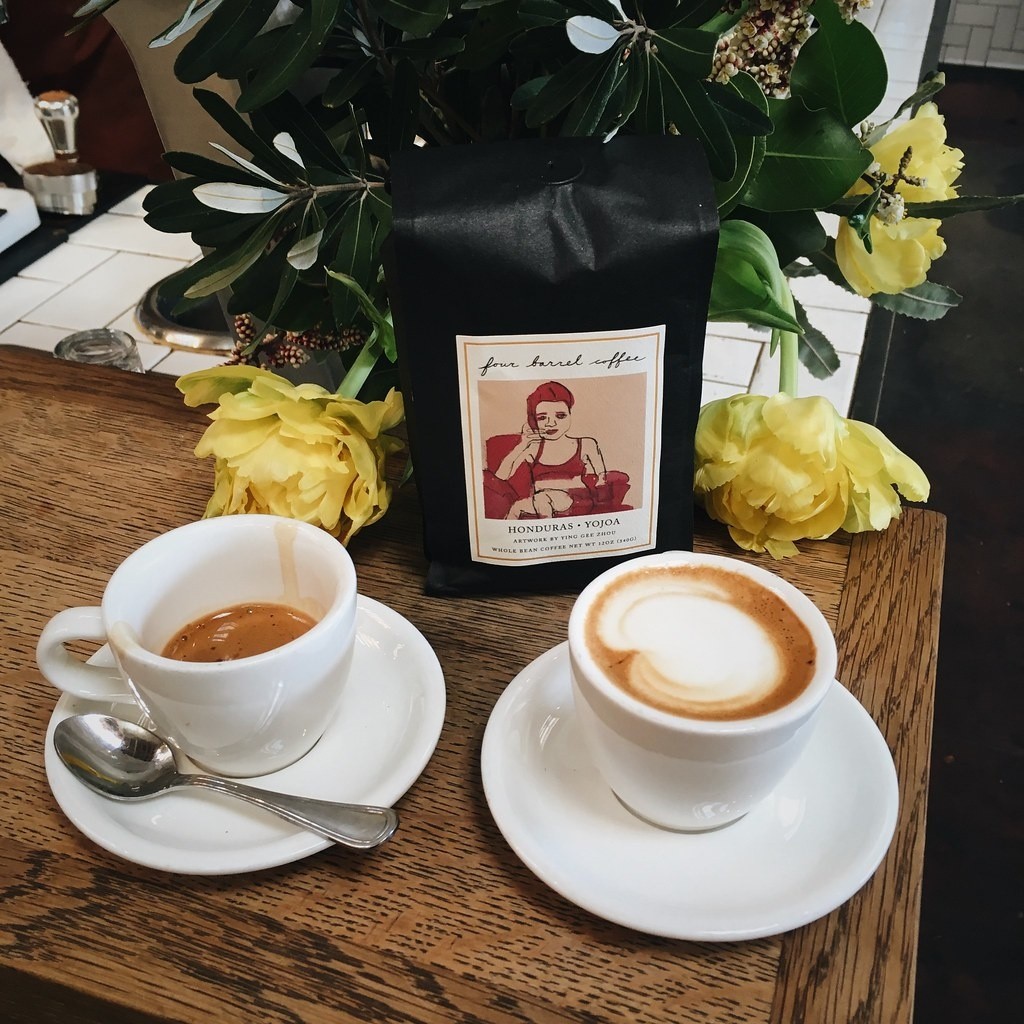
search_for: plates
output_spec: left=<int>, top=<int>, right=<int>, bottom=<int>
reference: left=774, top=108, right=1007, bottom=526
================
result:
left=481, top=638, right=901, bottom=942
left=44, top=591, right=447, bottom=875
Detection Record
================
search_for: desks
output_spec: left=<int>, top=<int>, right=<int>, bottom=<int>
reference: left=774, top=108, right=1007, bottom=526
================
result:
left=0, top=343, right=945, bottom=1024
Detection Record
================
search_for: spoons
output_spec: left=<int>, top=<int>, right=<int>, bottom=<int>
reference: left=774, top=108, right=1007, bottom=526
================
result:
left=51, top=714, right=395, bottom=848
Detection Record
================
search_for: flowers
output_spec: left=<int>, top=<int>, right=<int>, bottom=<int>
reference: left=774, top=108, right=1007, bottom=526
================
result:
left=61, top=0, right=1024, bottom=560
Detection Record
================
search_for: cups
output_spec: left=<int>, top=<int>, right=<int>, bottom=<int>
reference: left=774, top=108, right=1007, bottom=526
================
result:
left=36, top=513, right=359, bottom=777
left=570, top=548, right=838, bottom=832
left=50, top=327, right=146, bottom=376
